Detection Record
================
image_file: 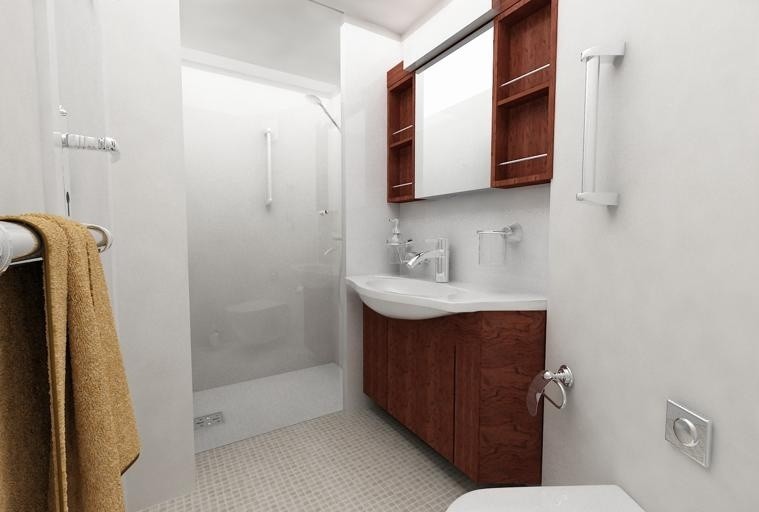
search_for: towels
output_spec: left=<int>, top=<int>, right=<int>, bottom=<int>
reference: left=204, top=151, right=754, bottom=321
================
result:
left=1, top=211, right=142, bottom=511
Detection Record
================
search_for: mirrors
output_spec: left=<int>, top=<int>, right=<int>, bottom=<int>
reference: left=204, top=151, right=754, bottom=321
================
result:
left=414, top=7, right=491, bottom=199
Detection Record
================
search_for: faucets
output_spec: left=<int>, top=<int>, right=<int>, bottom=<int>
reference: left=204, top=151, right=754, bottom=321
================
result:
left=323, top=246, right=337, bottom=256
left=406, top=238, right=449, bottom=282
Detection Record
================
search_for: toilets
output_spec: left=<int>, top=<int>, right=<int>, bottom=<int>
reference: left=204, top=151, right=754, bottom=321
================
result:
left=222, top=299, right=289, bottom=349
left=443, top=483, right=646, bottom=512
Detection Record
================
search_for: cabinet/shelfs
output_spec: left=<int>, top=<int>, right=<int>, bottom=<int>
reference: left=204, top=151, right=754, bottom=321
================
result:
left=491, top=0, right=553, bottom=189
left=359, top=302, right=545, bottom=488
left=387, top=60, right=415, bottom=202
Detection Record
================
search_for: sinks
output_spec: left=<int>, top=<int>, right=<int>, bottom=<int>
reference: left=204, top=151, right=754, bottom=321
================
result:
left=294, top=262, right=339, bottom=290
left=345, top=271, right=547, bottom=321
